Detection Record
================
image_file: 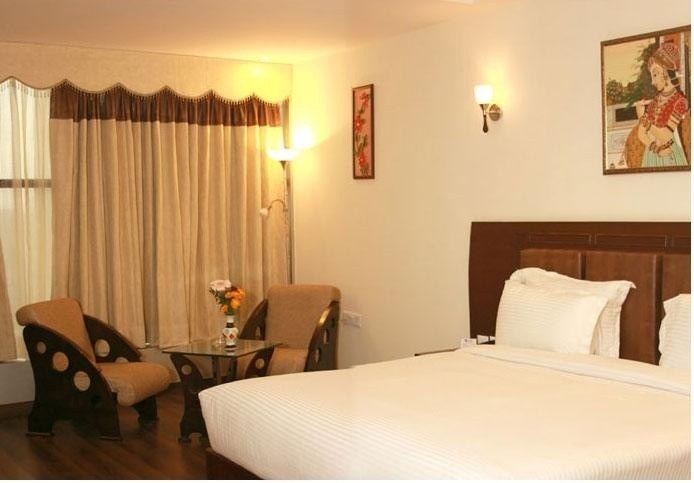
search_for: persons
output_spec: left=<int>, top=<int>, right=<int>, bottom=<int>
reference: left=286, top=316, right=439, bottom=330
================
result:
left=632, top=41, right=689, bottom=168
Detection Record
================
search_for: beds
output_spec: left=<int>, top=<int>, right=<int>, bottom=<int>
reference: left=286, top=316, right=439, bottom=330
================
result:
left=198, top=221, right=692, bottom=479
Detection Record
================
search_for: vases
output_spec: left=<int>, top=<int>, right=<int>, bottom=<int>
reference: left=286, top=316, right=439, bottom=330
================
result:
left=222, top=314, right=239, bottom=350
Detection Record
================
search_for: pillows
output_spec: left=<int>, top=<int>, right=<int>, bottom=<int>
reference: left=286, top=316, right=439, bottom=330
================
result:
left=494, top=267, right=691, bottom=372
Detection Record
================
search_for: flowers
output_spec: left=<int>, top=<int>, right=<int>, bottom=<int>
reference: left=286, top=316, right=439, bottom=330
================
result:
left=208, top=280, right=246, bottom=316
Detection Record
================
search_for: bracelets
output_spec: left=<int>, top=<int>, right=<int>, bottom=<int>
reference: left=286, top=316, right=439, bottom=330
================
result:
left=638, top=112, right=652, bottom=131
left=647, top=140, right=661, bottom=154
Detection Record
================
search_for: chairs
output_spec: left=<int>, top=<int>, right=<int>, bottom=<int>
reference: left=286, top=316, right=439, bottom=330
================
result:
left=226, top=284, right=342, bottom=382
left=16, top=296, right=170, bottom=441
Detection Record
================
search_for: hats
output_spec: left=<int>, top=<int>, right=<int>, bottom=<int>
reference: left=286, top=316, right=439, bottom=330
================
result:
left=648, top=45, right=680, bottom=71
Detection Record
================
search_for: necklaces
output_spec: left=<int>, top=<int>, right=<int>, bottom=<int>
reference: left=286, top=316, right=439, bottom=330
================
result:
left=659, top=86, right=677, bottom=96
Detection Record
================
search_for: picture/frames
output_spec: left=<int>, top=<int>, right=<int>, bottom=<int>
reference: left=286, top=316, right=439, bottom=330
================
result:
left=352, top=83, right=375, bottom=179
left=600, top=24, right=691, bottom=175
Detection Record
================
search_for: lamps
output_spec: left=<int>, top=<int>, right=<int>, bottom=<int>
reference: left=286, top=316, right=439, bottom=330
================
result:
left=259, top=199, right=288, bottom=219
left=474, top=85, right=501, bottom=132
